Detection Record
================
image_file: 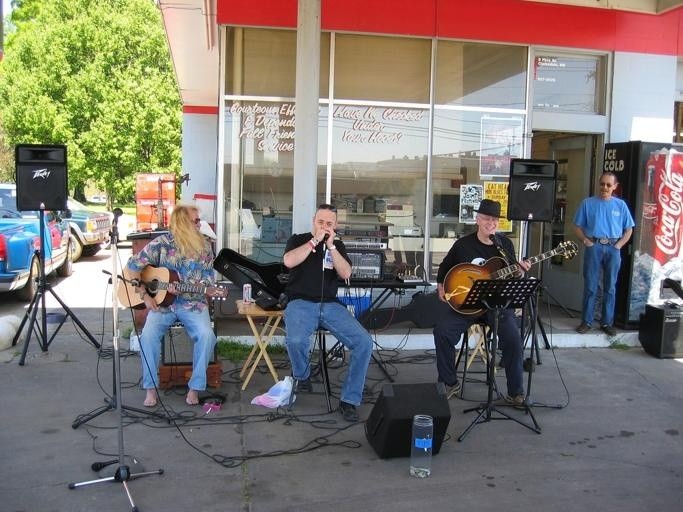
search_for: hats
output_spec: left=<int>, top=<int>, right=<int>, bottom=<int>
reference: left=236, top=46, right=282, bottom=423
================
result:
left=473, top=199, right=506, bottom=219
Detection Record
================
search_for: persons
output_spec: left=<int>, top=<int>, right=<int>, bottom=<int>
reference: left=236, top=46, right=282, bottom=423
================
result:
left=282, top=204, right=373, bottom=422
left=432, top=199, right=531, bottom=410
left=574, top=172, right=636, bottom=336
left=127, top=204, right=229, bottom=407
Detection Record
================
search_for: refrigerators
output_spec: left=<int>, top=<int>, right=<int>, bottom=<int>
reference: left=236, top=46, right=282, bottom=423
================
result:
left=602, top=139, right=683, bottom=331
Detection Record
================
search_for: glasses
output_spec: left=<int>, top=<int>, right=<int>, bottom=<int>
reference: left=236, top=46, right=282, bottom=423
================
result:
left=191, top=218, right=201, bottom=225
left=599, top=182, right=617, bottom=188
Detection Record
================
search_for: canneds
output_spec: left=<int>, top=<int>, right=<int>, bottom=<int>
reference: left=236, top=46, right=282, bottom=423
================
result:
left=638, top=150, right=683, bottom=266
left=242, top=283, right=252, bottom=306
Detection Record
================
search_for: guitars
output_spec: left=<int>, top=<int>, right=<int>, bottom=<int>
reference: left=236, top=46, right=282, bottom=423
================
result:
left=443, top=241, right=579, bottom=320
left=118, top=264, right=229, bottom=310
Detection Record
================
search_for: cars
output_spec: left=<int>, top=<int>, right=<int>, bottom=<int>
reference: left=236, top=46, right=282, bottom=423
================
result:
left=0, top=185, right=112, bottom=298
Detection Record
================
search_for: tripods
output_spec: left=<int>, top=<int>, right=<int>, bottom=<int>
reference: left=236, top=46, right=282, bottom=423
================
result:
left=13, top=212, right=100, bottom=366
left=69, top=241, right=164, bottom=512
left=459, top=307, right=542, bottom=441
left=498, top=245, right=574, bottom=429
left=71, top=285, right=166, bottom=429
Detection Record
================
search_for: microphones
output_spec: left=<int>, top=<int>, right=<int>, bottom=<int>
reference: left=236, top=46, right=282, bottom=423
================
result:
left=488, top=233, right=500, bottom=246
left=323, top=233, right=330, bottom=241
left=91, top=459, right=119, bottom=472
left=111, top=208, right=124, bottom=244
left=132, top=277, right=142, bottom=288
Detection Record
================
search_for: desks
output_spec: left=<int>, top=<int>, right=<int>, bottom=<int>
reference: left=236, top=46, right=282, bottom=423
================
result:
left=232, top=299, right=289, bottom=391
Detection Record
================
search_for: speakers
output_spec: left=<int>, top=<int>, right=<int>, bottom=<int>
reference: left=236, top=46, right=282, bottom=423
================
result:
left=506, top=158, right=558, bottom=222
left=364, top=383, right=451, bottom=459
left=638, top=302, right=683, bottom=358
left=15, top=143, right=68, bottom=211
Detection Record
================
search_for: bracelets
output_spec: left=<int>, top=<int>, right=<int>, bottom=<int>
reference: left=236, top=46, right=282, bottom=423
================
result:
left=329, top=245, right=337, bottom=251
left=140, top=292, right=147, bottom=300
left=307, top=237, right=320, bottom=253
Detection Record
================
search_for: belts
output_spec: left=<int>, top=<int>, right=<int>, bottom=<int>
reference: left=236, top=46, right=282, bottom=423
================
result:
left=589, top=238, right=618, bottom=245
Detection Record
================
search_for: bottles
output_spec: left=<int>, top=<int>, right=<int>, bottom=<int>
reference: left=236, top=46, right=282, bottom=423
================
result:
left=409, top=414, right=434, bottom=478
left=638, top=165, right=658, bottom=257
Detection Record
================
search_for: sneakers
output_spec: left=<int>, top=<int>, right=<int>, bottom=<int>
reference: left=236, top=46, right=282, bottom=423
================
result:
left=339, top=400, right=359, bottom=421
left=505, top=391, right=526, bottom=410
left=600, top=325, right=616, bottom=336
left=297, top=377, right=314, bottom=394
left=445, top=380, right=462, bottom=400
left=576, top=323, right=591, bottom=334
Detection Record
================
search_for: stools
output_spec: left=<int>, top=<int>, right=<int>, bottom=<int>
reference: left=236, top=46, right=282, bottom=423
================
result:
left=288, top=327, right=345, bottom=412
left=455, top=314, right=499, bottom=397
